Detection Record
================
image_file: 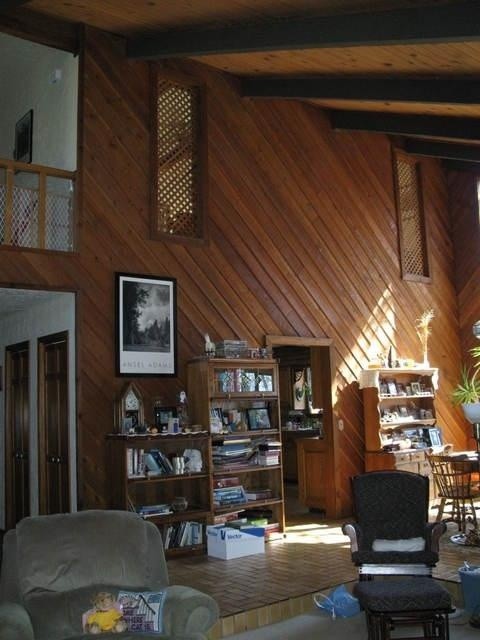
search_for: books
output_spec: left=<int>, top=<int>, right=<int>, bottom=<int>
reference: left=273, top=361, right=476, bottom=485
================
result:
left=127, top=434, right=284, bottom=550
left=448, top=449, right=479, bottom=462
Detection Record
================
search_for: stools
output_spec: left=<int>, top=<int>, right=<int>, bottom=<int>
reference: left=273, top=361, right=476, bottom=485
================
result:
left=352, top=579, right=455, bottom=640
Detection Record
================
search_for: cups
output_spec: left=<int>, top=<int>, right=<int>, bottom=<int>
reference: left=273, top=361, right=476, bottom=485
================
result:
left=172, top=456, right=185, bottom=476
left=167, top=417, right=179, bottom=433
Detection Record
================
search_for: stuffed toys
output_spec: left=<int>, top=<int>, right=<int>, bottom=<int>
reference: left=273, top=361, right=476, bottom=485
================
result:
left=83, top=590, right=129, bottom=636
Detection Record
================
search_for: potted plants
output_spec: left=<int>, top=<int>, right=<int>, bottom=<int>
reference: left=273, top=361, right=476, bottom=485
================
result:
left=449, top=346, right=480, bottom=424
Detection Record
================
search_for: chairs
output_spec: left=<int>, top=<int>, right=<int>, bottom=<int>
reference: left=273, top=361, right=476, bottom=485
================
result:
left=1, top=510, right=220, bottom=640
left=423, top=444, right=480, bottom=533
left=340, top=470, right=448, bottom=611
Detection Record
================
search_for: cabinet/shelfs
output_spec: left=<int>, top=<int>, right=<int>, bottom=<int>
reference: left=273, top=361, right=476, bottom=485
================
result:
left=362, top=369, right=454, bottom=508
left=283, top=435, right=319, bottom=478
left=106, top=357, right=286, bottom=562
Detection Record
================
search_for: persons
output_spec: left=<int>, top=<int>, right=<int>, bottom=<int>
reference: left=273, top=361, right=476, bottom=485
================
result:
left=162, top=412, right=171, bottom=423
left=173, top=420, right=180, bottom=433
left=255, top=412, right=265, bottom=428
left=124, top=419, right=131, bottom=433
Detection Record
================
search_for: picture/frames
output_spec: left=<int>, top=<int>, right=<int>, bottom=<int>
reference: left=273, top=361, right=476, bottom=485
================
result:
left=14, top=108, right=32, bottom=175
left=113, top=271, right=178, bottom=379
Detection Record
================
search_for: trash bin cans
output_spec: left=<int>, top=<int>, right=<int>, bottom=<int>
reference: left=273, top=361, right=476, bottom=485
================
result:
left=457, top=565, right=480, bottom=614
